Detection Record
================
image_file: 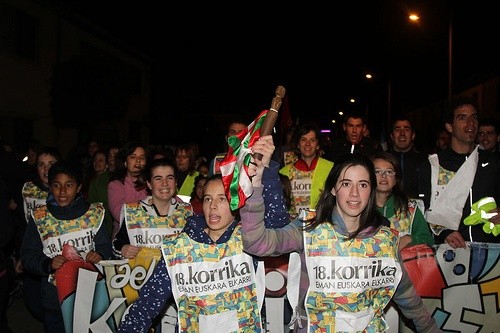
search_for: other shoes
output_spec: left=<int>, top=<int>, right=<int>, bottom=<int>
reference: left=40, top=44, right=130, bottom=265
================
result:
left=8, top=279, right=24, bottom=307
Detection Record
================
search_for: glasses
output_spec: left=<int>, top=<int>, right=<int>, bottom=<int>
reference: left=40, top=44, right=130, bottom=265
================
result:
left=374, top=168, right=396, bottom=176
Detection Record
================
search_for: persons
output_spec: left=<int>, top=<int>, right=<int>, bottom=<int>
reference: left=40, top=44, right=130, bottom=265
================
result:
left=118, top=134, right=291, bottom=333
left=210, top=112, right=385, bottom=228
left=0, top=139, right=210, bottom=333
left=377, top=102, right=500, bottom=251
left=241, top=157, right=441, bottom=333
